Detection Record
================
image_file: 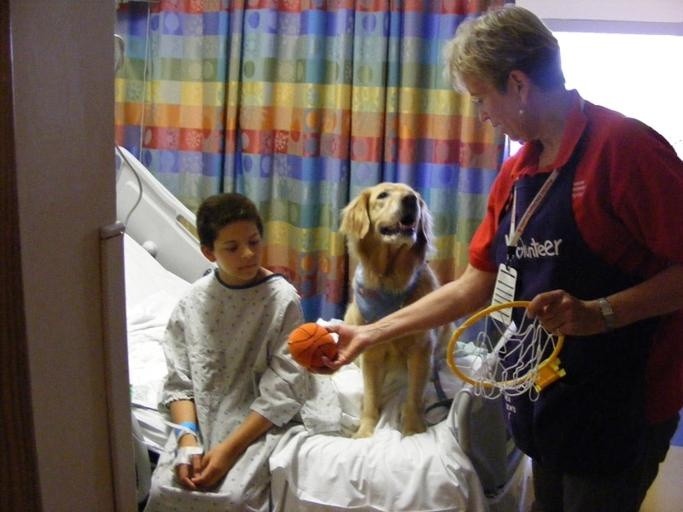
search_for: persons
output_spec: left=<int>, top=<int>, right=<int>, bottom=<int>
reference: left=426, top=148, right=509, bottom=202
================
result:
left=144, top=188, right=308, bottom=512
left=285, top=1, right=683, bottom=512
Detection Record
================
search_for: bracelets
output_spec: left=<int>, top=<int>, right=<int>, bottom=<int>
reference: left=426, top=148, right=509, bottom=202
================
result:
left=172, top=422, right=198, bottom=439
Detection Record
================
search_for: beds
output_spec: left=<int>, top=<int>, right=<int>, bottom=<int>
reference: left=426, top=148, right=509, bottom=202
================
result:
left=113, top=144, right=533, bottom=512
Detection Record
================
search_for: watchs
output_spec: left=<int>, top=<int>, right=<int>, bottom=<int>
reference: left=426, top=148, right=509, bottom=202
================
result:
left=594, top=296, right=617, bottom=335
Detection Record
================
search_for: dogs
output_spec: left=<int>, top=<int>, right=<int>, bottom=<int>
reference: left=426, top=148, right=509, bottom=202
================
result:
left=339, top=182, right=458, bottom=438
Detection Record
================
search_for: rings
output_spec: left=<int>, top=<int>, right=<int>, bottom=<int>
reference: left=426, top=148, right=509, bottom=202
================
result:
left=557, top=325, right=563, bottom=337
